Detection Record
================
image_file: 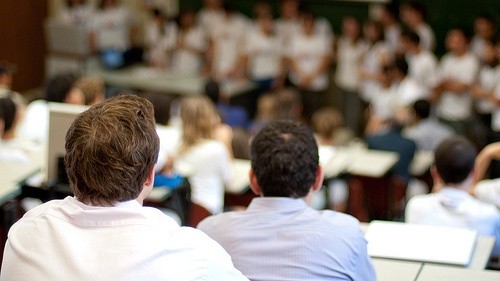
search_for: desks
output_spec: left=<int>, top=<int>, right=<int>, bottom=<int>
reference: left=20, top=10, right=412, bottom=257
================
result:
left=364, top=217, right=499, bottom=281
left=96, top=65, right=258, bottom=123
left=336, top=146, right=399, bottom=224
left=224, top=162, right=257, bottom=207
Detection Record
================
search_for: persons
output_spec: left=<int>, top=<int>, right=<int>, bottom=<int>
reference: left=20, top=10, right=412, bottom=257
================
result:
left=0, top=98, right=47, bottom=213
left=0, top=59, right=32, bottom=105
left=1, top=93, right=254, bottom=281
left=193, top=118, right=379, bottom=281
left=43, top=0, right=500, bottom=148
left=464, top=139, right=499, bottom=210
left=307, top=108, right=373, bottom=181
left=158, top=94, right=253, bottom=216
left=401, top=135, right=500, bottom=261
left=393, top=97, right=464, bottom=195
